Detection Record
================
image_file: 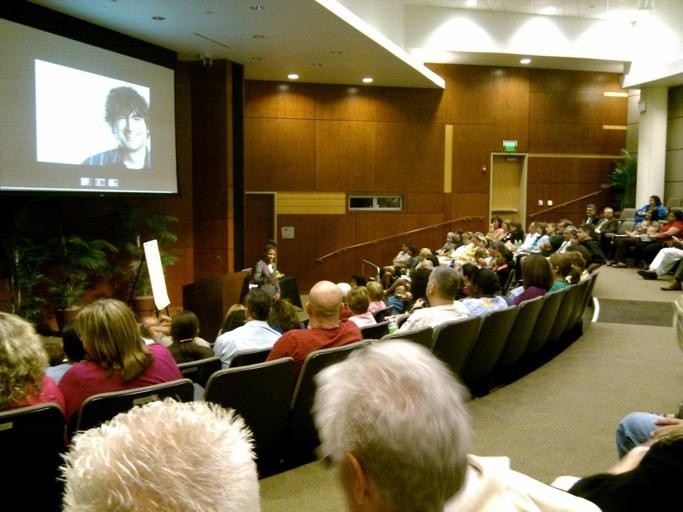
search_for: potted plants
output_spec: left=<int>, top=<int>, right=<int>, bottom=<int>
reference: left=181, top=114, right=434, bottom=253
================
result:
left=122, top=213, right=178, bottom=323
left=45, top=235, right=119, bottom=331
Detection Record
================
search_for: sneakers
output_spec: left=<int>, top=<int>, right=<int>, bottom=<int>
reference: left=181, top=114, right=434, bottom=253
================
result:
left=637, top=270, right=658, bottom=280
left=660, top=284, right=681, bottom=291
left=606, top=260, right=627, bottom=267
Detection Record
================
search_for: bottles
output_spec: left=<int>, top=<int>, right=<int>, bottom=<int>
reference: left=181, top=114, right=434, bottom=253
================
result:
left=387, top=317, right=399, bottom=334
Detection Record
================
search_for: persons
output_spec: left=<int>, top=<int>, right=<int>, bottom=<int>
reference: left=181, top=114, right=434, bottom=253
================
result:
left=311, top=340, right=601, bottom=512
left=1, top=215, right=605, bottom=439
left=80, top=87, right=151, bottom=168
left=625, top=196, right=683, bottom=291
left=582, top=203, right=618, bottom=246
left=551, top=402, right=683, bottom=512
left=59, top=398, right=260, bottom=512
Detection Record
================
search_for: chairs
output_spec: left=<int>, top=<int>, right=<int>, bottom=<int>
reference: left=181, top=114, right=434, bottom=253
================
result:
left=604, top=207, right=683, bottom=264
